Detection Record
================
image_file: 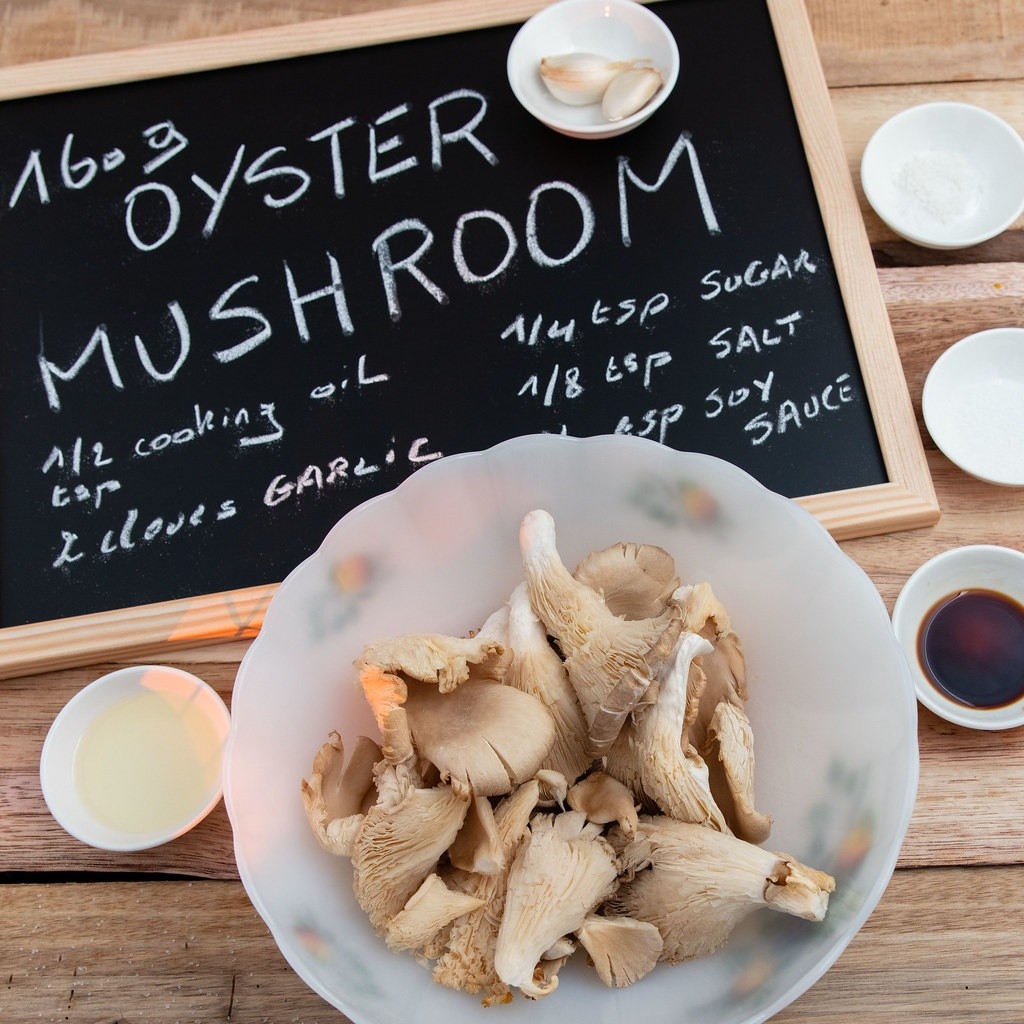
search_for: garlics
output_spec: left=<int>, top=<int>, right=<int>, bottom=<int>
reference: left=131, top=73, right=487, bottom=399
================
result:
left=536, top=52, right=661, bottom=122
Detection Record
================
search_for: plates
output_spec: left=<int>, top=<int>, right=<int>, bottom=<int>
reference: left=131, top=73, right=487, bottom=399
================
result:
left=889, top=544, right=1024, bottom=731
left=506, top=0, right=680, bottom=141
left=226, top=431, right=921, bottom=1024
left=39, top=664, right=232, bottom=853
left=860, top=101, right=1024, bottom=251
left=921, top=328, right=1024, bottom=489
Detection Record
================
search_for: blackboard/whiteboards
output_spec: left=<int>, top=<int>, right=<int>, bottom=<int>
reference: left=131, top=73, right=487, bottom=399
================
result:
left=0, top=0, right=943, bottom=681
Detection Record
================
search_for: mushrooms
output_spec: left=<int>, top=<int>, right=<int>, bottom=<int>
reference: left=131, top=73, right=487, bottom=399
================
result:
left=301, top=511, right=837, bottom=1005
